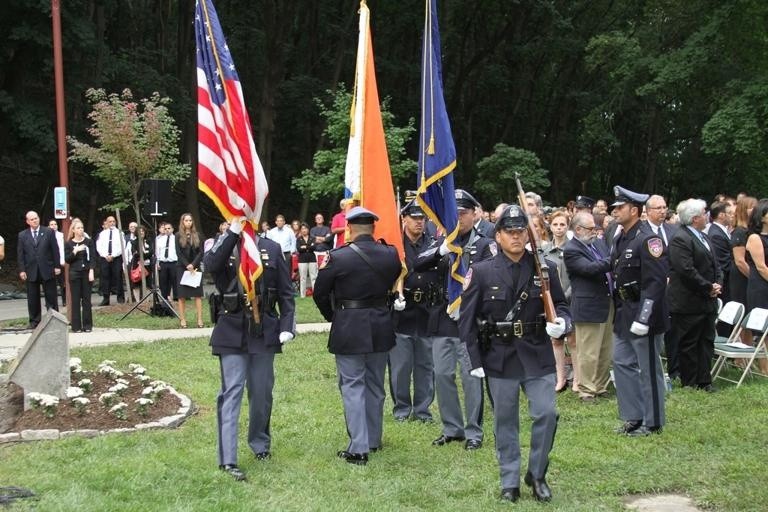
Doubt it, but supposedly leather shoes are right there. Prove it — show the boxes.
[99,300,109,306]
[626,425,663,437]
[613,419,642,434]
[27,323,37,329]
[337,450,368,466]
[524,470,552,502]
[257,452,271,462]
[465,439,482,451]
[496,486,520,504]
[432,434,465,446]
[220,465,244,480]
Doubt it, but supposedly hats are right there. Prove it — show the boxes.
[454,189,479,210]
[404,190,417,204]
[494,205,528,232]
[345,206,379,224]
[609,185,649,206]
[403,200,426,217]
[573,196,595,209]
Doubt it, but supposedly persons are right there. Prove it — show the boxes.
[730,197,758,369]
[413,189,497,450]
[157,224,179,302]
[125,222,139,244]
[473,204,496,240]
[460,205,575,504]
[47,220,66,306]
[267,214,296,273]
[665,210,677,224]
[64,221,96,333]
[744,198,767,375]
[543,210,579,392]
[204,220,296,480]
[297,223,318,298]
[17,211,61,330]
[525,190,541,216]
[596,200,613,222]
[727,205,737,237]
[388,199,436,427]
[527,216,548,251]
[96,216,126,306]
[175,213,204,328]
[0,234,6,258]
[313,207,402,466]
[260,222,271,239]
[610,185,667,438]
[737,191,748,204]
[292,220,300,235]
[332,199,346,249]
[310,213,331,255]
[668,198,724,395]
[155,222,165,238]
[705,194,725,223]
[642,195,678,246]
[708,202,735,338]
[130,226,152,302]
[576,196,595,215]
[563,212,614,405]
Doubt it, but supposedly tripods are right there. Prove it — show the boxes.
[120,217,181,323]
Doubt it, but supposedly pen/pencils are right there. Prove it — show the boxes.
[193,268,198,275]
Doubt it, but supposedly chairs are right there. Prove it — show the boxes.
[712,308,768,389]
[708,301,746,375]
[714,298,722,325]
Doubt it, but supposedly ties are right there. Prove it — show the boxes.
[165,236,169,258]
[108,230,112,256]
[33,231,38,245]
[588,243,614,297]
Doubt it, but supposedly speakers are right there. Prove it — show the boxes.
[142,179,172,219]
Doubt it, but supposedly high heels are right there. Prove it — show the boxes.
[554,379,568,393]
[180,318,187,328]
[197,319,204,328]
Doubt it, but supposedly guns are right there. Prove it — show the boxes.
[514,172,559,324]
[394,185,404,301]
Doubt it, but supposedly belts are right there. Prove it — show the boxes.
[614,288,629,302]
[488,321,536,336]
[333,295,387,309]
[403,288,426,303]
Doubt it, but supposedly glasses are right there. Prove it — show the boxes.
[579,225,593,230]
[650,207,668,211]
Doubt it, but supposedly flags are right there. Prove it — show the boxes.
[195,0,269,324]
[344,0,408,295]
[417,1,466,314]
